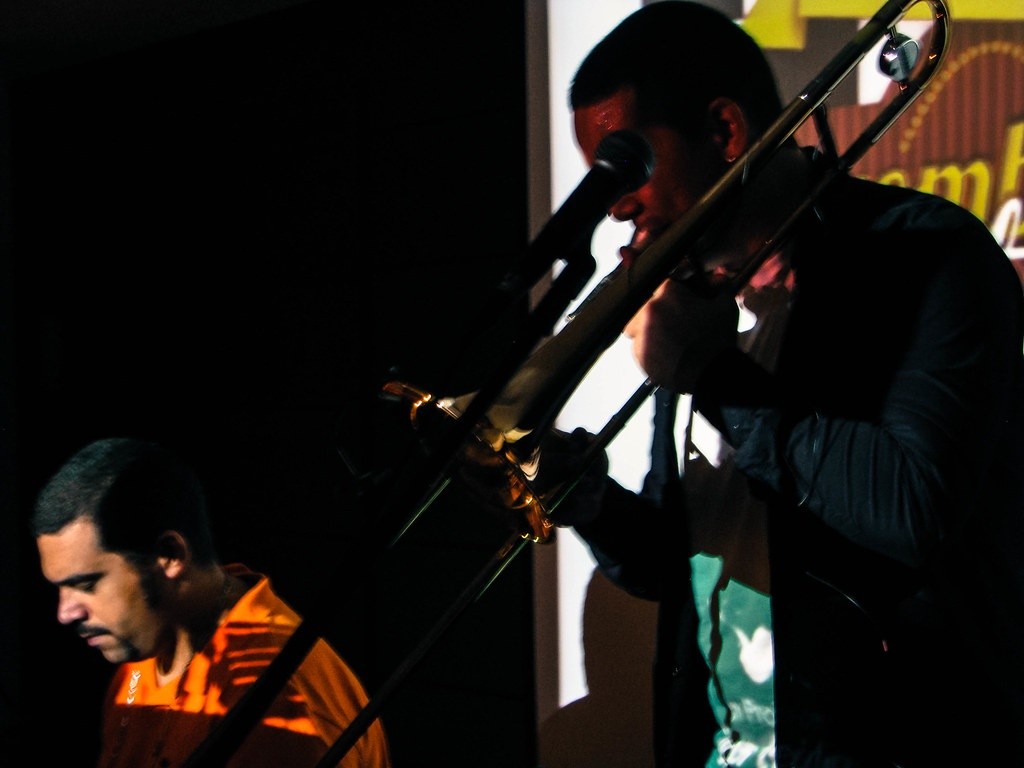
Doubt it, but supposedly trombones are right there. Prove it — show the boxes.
[183,0,955,768]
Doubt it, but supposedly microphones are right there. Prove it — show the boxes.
[500,129,657,304]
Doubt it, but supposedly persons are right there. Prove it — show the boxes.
[35,439,389,767]
[536,0,1024,768]
